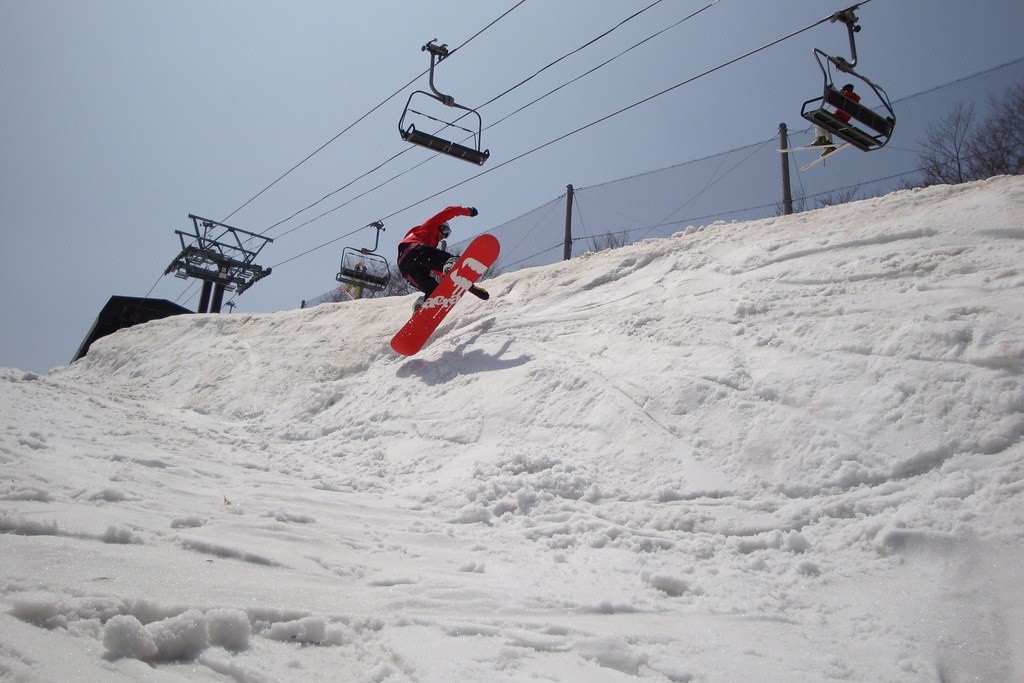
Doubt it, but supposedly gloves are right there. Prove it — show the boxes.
[468,207,478,217]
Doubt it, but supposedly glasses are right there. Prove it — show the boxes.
[440,224,452,238]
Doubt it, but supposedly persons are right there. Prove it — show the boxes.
[396,207,489,311]
[345,261,363,291]
[807,84,861,157]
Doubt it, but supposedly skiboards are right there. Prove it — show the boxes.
[775,142,851,171]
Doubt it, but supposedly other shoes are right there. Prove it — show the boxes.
[443,257,459,276]
[412,296,425,312]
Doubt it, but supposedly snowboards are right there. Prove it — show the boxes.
[391,233,502,357]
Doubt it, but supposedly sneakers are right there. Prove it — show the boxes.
[820,147,836,157]
[810,136,826,146]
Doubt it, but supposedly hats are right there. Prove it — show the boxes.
[442,221,450,229]
[842,84,854,93]
